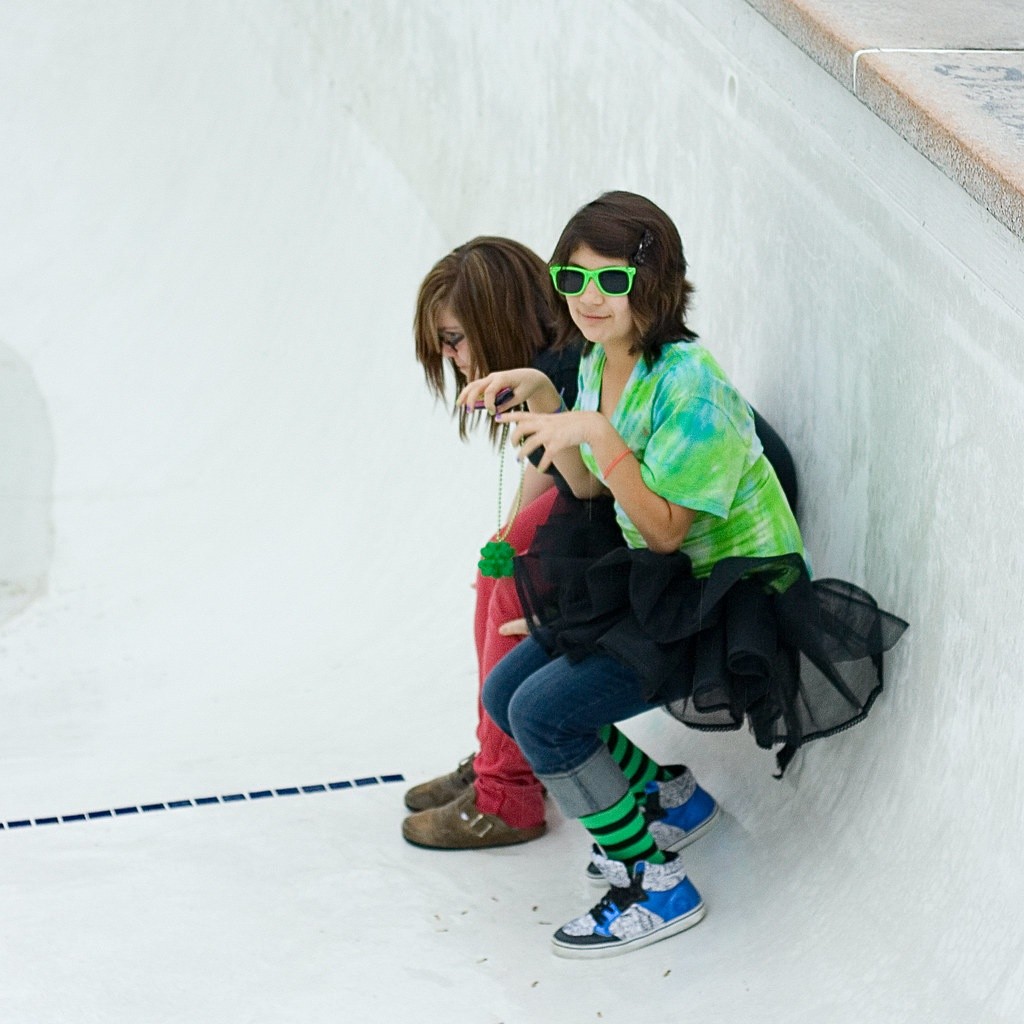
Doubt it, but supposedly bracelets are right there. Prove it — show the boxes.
[597,445,638,484]
[548,391,567,427]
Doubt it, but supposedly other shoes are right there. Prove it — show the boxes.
[401,753,549,852]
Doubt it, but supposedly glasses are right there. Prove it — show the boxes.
[440,335,465,353]
[550,264,636,296]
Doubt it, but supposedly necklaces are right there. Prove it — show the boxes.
[476,387,533,582]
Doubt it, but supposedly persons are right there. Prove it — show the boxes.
[453,186,815,962]
[396,231,803,856]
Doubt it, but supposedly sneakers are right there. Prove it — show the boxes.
[551,849,705,957]
[583,764,721,888]
